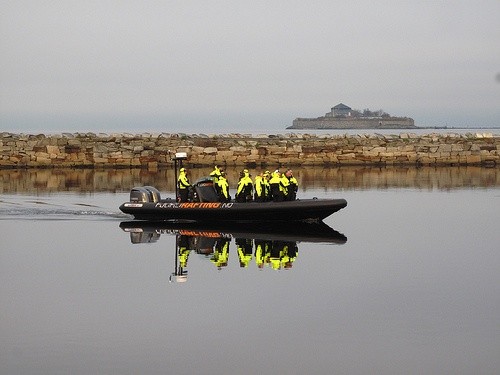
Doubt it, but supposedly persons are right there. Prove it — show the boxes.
[177,168,189,202]
[208,164,299,201]
[178,235,191,268]
[209,237,299,270]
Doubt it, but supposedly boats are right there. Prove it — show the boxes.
[119,152,348,221]
